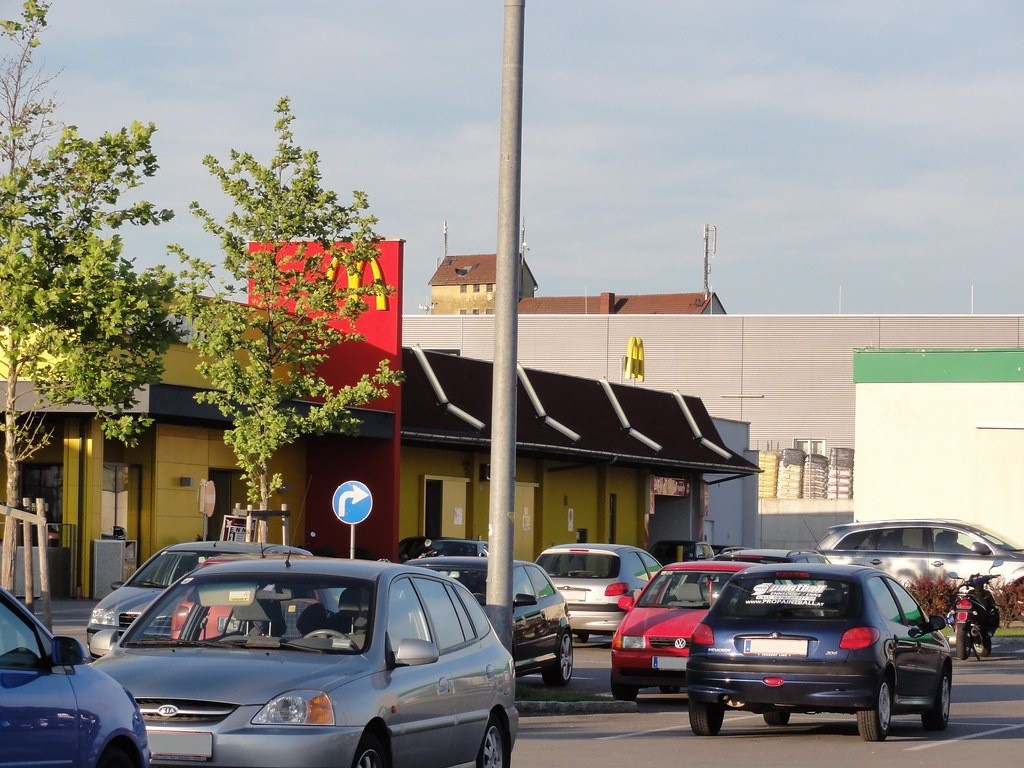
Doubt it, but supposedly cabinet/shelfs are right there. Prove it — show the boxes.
[93,539,137,600]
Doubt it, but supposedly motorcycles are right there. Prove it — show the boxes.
[948,558,1004,661]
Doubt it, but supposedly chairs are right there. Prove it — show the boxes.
[674,583,703,602]
[339,587,372,635]
[822,590,840,604]
[219,590,281,639]
[570,559,586,571]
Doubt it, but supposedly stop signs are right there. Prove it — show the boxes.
[204,481,215,518]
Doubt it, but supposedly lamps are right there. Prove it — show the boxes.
[180,477,194,486]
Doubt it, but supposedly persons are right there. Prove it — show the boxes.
[938,528,971,552]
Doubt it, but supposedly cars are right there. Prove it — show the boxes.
[171,551,326,641]
[685,563,955,742]
[604,561,766,701]
[814,518,1024,622]
[398,536,488,559]
[646,539,713,561]
[88,556,518,768]
[86,541,338,648]
[402,557,573,686]
[0,588,154,768]
[709,544,751,554]
[522,543,663,641]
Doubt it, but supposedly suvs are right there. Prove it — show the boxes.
[697,547,832,603]
